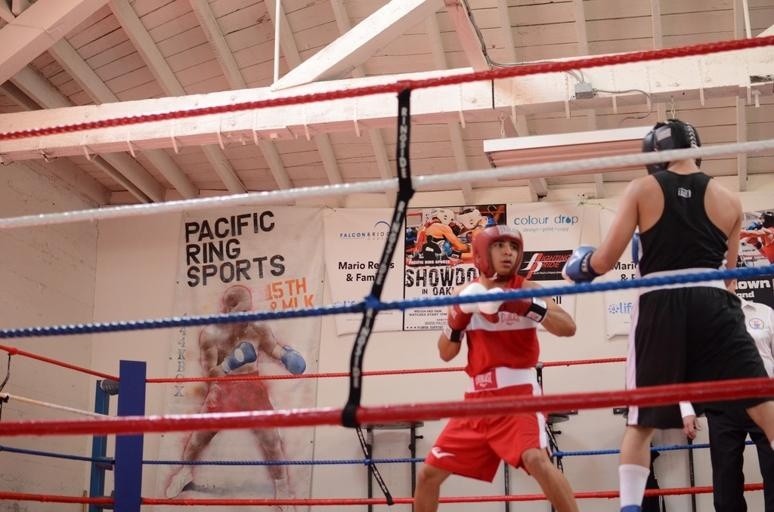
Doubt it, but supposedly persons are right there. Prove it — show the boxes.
[738,210,774,268]
[406,205,505,264]
[677,264,773,512]
[564,118,774,512]
[406,224,580,511]
[165,286,305,512]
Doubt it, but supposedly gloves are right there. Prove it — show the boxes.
[746,236,758,244]
[279,345,307,375]
[561,246,601,284]
[224,341,258,370]
[746,221,758,230]
[443,282,488,343]
[478,286,549,324]
[740,230,747,240]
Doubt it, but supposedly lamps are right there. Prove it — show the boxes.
[482,95,676,183]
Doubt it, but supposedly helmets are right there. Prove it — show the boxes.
[457,207,482,230]
[642,118,702,176]
[472,224,524,282]
[758,211,774,227]
[433,209,455,226]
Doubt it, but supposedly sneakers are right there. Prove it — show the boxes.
[275,494,296,512]
[165,471,194,499]
